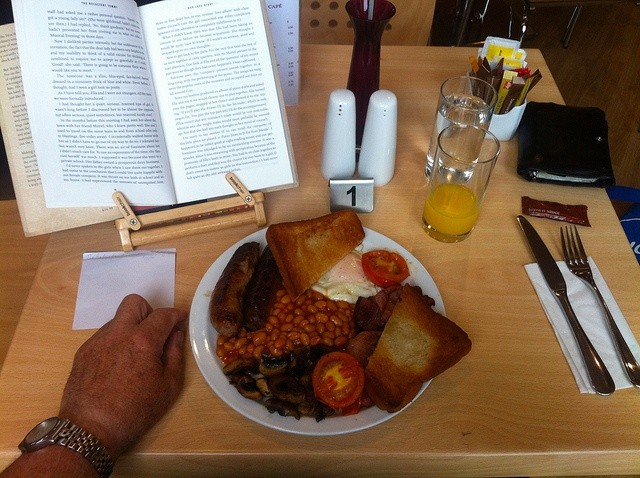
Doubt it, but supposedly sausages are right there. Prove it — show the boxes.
[239,242,283,333]
[208,243,261,339]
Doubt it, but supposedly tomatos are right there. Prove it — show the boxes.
[358,247,411,288]
[312,351,364,409]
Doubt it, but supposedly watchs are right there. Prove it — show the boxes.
[18,417,114,478]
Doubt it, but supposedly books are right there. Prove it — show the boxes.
[517,102,616,188]
[12,0,299,210]
[0,22,164,237]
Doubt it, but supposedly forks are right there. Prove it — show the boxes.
[560,225,640,389]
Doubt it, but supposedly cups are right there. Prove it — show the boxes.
[420,122,500,244]
[484,98,530,141]
[423,75,498,186]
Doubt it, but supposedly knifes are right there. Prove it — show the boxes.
[516,214,615,396]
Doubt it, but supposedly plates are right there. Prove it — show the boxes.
[188,225,446,438]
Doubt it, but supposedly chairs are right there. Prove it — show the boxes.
[431,0,492,48]
[502,0,587,49]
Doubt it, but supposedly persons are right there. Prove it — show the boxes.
[0,294,187,478]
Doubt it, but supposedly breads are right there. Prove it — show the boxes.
[265,209,366,303]
[362,283,474,410]
